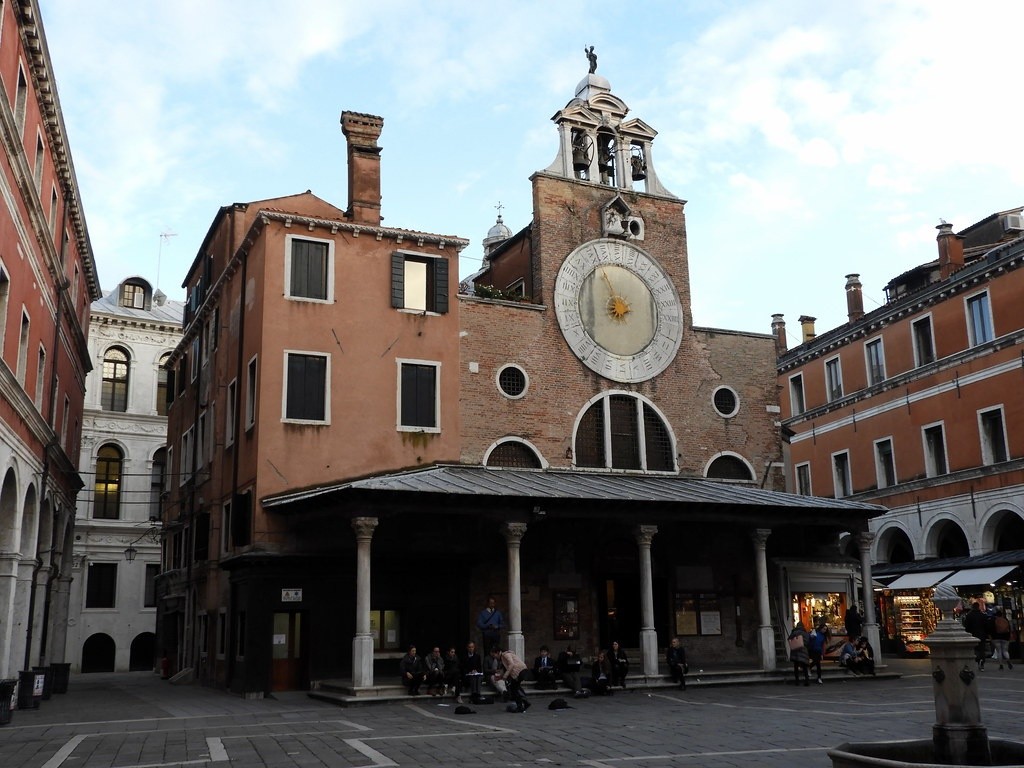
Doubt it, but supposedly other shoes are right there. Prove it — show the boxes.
[818,678,823,684]
[999,665,1003,669]
[408,691,415,695]
[807,666,812,677]
[456,696,464,704]
[1009,664,1013,670]
[981,668,985,672]
[415,691,420,694]
[524,702,530,710]
[452,686,456,695]
[428,689,436,695]
[439,690,443,695]
[513,706,524,713]
[979,660,983,669]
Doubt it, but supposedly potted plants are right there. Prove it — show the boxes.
[518,296,532,304]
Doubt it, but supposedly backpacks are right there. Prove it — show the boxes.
[995,615,1010,634]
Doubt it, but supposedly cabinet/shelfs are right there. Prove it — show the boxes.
[896,598,923,631]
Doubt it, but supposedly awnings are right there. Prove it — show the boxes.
[883,566,1017,589]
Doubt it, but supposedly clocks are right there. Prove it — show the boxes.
[553,237,683,384]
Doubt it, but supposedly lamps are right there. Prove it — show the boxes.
[125,527,158,565]
[566,447,573,461]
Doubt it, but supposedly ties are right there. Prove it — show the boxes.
[543,659,545,667]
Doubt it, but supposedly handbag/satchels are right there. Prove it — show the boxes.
[788,630,804,651]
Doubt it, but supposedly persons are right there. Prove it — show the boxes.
[789,621,830,685]
[965,603,1013,672]
[534,645,589,698]
[586,46,597,73]
[841,604,875,675]
[400,598,531,713]
[592,641,629,695]
[666,638,688,691]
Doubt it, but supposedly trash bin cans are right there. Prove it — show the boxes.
[18,670,46,709]
[0,679,19,724]
[32,667,56,700]
[50,662,71,694]
